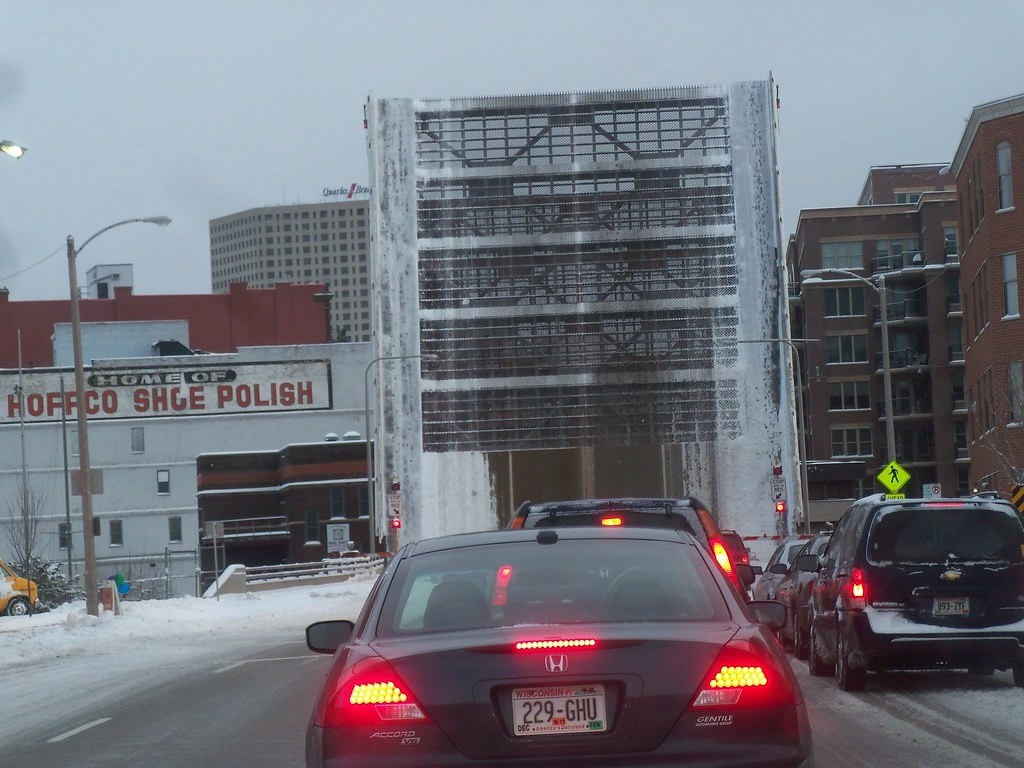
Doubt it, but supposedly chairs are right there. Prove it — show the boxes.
[422,564,697,624]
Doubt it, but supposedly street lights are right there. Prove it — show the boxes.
[364,354,441,552]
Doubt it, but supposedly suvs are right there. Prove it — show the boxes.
[809,492,1024,692]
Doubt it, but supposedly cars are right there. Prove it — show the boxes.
[0,559,38,617]
[509,496,765,625]
[752,531,836,660]
[68,215,171,618]
[305,528,814,768]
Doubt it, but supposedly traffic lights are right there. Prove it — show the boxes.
[390,481,400,491]
[391,519,399,527]
